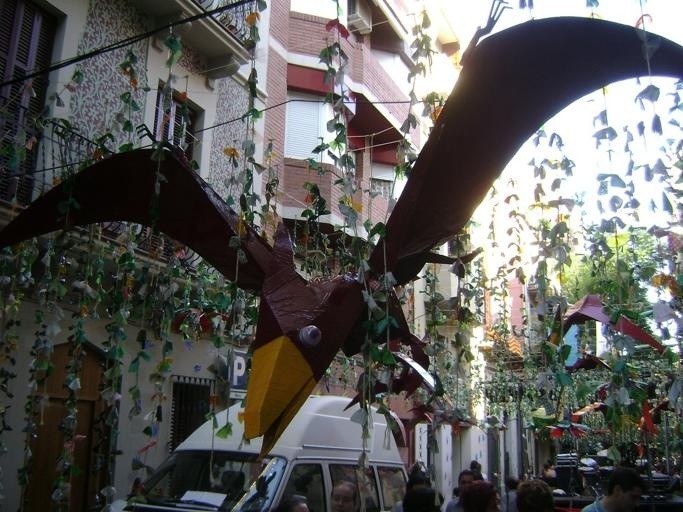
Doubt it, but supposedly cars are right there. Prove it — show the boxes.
[555,452,681,488]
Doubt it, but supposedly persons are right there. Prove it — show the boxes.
[581,467,648,512]
[330,481,361,512]
[270,495,311,512]
[391,460,557,512]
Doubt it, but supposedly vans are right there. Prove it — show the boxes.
[123,395,409,512]
